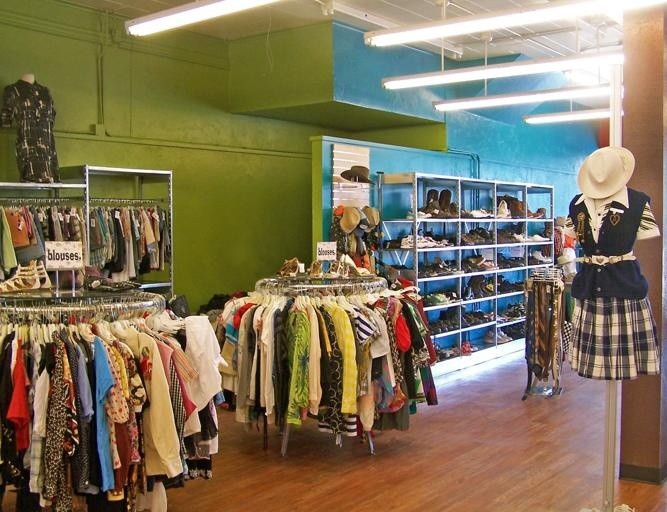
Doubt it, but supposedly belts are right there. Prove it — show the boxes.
[521,281,560,401]
[584,249,637,266]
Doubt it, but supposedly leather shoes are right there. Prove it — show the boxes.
[83,274,142,292]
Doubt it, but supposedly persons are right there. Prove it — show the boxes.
[1,72,61,183]
[567,145,660,381]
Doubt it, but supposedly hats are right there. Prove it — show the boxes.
[577,145,636,200]
[341,165,376,184]
[554,216,567,231]
[339,206,380,234]
[556,255,573,265]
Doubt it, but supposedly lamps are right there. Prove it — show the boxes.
[361,0,624,125]
[124,0,277,38]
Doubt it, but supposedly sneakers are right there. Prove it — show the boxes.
[383,227,551,249]
[400,251,553,359]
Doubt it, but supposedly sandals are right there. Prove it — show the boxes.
[0,260,53,294]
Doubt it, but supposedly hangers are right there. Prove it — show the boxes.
[0,197,69,211]
[0,298,185,346]
[254,279,366,310]
[90,197,158,211]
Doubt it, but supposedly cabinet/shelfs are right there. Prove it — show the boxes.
[376,171,556,381]
[0,163,175,303]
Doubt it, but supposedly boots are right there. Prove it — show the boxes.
[406,190,546,219]
